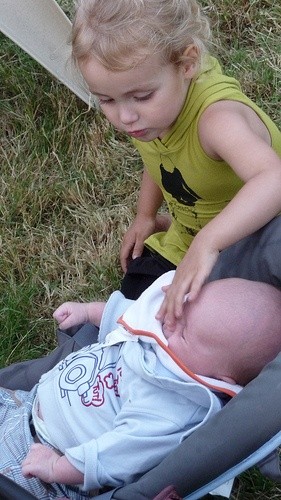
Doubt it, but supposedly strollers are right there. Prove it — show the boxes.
[0,211,281,500]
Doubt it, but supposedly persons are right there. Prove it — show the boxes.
[66,0,281,328]
[1,276,281,499]
